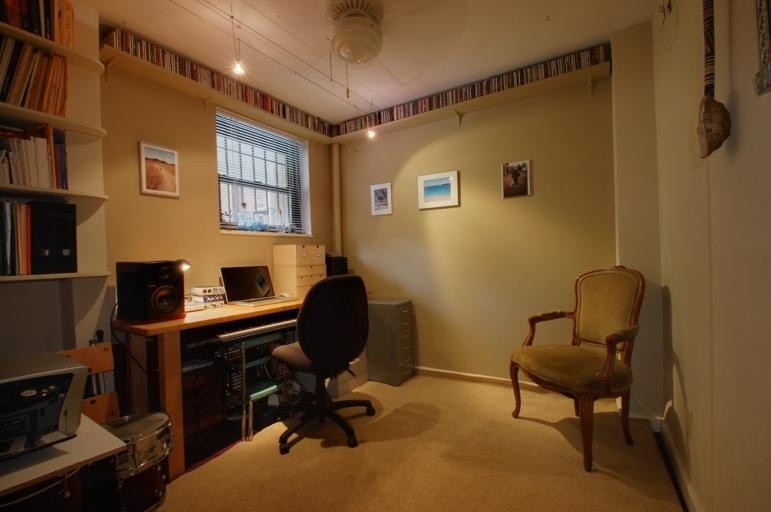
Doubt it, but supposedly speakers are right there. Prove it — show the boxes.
[116,260,185,325]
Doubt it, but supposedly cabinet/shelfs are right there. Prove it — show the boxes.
[367,297,414,387]
[274,243,325,296]
[1,0,113,403]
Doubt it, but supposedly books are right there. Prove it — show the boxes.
[105,26,332,137]
[338,44,606,136]
[2,1,78,275]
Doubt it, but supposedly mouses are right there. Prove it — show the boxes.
[280,293,290,298]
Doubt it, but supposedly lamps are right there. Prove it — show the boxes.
[176,257,192,272]
[325,1,386,66]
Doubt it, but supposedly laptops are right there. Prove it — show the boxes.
[220,265,291,307]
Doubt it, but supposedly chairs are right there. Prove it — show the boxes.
[270,272,378,451]
[506,263,645,472]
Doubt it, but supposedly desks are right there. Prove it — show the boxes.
[0,412,129,512]
[111,294,304,480]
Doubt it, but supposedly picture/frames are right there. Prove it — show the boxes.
[371,183,394,216]
[417,170,459,211]
[139,141,180,198]
